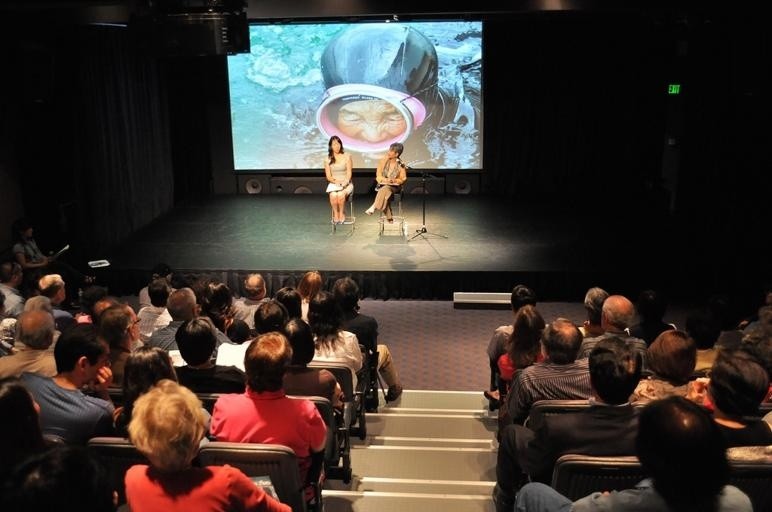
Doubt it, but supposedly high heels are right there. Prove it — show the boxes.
[339,214,346,224]
[364,206,375,216]
[387,210,393,224]
[332,215,339,225]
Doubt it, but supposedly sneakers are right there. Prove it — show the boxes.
[484,391,500,403]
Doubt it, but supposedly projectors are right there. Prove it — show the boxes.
[159,0,251,59]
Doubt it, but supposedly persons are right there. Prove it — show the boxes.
[2,262,25,317]
[512,394,751,511]
[36,275,73,330]
[324,137,354,225]
[274,287,303,319]
[13,324,118,484]
[74,286,107,322]
[714,289,772,359]
[298,272,322,326]
[1,384,71,511]
[308,291,363,400]
[13,221,81,308]
[167,270,188,289]
[631,329,709,407]
[487,285,550,367]
[89,299,145,351]
[199,282,251,344]
[702,352,772,447]
[232,274,272,334]
[138,264,176,309]
[210,331,328,495]
[5,443,122,511]
[496,319,595,422]
[492,336,648,511]
[332,277,403,413]
[278,317,346,428]
[366,141,407,224]
[123,379,291,511]
[241,301,291,371]
[135,279,172,344]
[22,296,62,348]
[0,309,58,378]
[146,288,234,357]
[484,305,546,402]
[579,288,629,339]
[630,290,677,346]
[193,277,207,305]
[111,345,208,439]
[90,304,142,387]
[174,317,247,395]
[1,293,20,355]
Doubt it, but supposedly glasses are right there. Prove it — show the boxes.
[132,318,141,326]
[10,261,17,276]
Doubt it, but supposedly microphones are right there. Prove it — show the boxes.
[396,159,404,168]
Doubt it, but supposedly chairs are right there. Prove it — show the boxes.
[196,442,325,511]
[85,387,127,407]
[727,445,772,512]
[284,393,353,484]
[550,452,644,502]
[85,435,146,506]
[359,344,378,414]
[41,433,67,449]
[306,359,371,442]
[193,391,224,417]
[522,398,591,440]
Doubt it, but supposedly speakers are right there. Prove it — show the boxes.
[446,169,489,195]
[272,172,446,194]
[236,171,270,195]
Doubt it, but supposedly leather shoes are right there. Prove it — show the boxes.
[387,385,403,401]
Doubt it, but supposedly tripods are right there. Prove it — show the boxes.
[407,168,448,242]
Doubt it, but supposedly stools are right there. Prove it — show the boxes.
[376,182,406,241]
[330,181,356,235]
